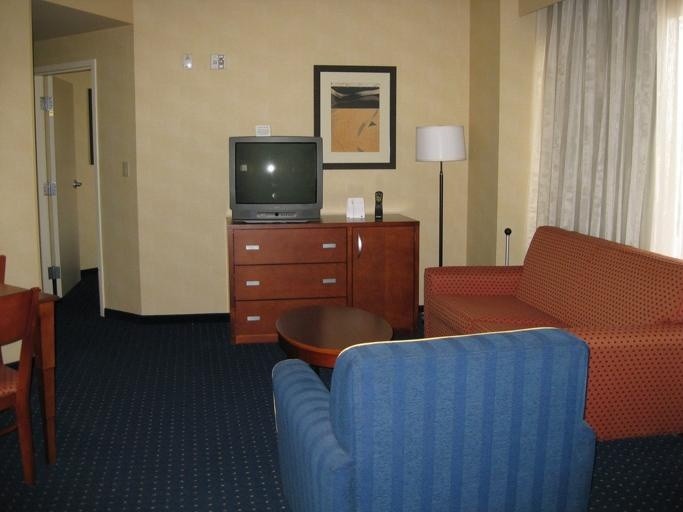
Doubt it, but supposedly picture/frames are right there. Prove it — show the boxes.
[313,64,396,170]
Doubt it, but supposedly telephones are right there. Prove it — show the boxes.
[375,191,383,222]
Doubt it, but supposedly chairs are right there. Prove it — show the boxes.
[0,286,41,484]
[0,255,7,284]
[271,326,598,511]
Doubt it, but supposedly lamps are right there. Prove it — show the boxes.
[415,126,466,267]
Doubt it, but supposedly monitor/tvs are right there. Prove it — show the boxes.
[228,136,324,224]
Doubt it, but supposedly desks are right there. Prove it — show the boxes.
[0,283,61,465]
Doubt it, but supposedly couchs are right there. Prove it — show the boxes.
[425,224,683,441]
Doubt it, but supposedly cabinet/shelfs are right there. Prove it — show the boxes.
[225,212,420,344]
